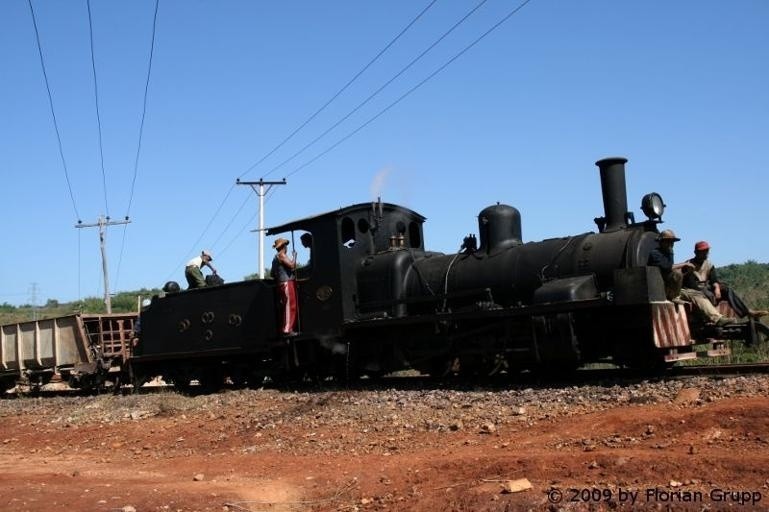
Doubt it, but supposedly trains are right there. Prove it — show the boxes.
[1,156,738,397]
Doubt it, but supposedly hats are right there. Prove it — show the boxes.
[201,250,215,261]
[272,238,290,249]
[694,241,712,253]
[654,229,680,242]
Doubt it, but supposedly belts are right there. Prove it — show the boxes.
[186,265,197,269]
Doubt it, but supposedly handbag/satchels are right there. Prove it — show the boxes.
[204,274,224,287]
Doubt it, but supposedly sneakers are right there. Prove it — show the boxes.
[281,329,297,336]
[716,316,736,326]
[747,308,769,319]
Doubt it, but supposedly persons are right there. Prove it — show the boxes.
[647,229,738,328]
[184,249,216,290]
[272,237,303,337]
[681,241,768,320]
